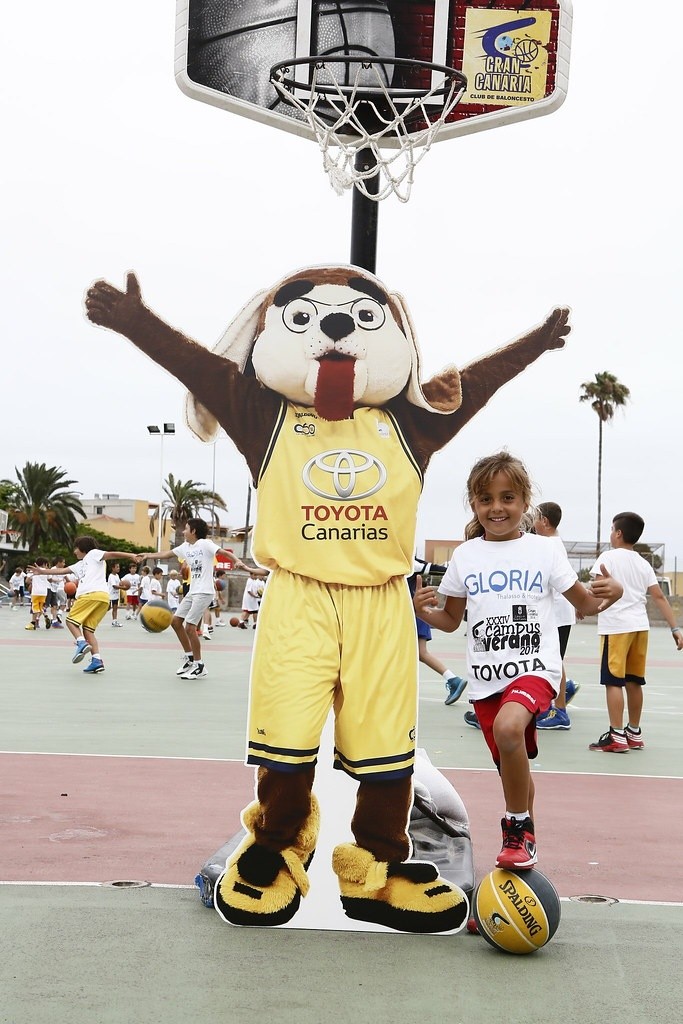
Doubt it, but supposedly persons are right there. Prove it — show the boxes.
[0,554,265,640]
[130,518,270,680]
[412,452,624,936]
[575,511,683,753]
[25,536,144,673]
[405,502,582,731]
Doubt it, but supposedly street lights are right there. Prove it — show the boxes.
[147,423,175,568]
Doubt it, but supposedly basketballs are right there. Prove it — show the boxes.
[471,865,562,954]
[229,617,240,626]
[139,599,174,634]
[176,585,184,594]
[214,579,225,591]
[121,580,131,590]
[64,582,78,594]
[258,588,264,596]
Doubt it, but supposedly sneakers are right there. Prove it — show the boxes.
[467,919,480,934]
[464,711,481,729]
[536,706,571,729]
[494,816,537,869]
[72,641,92,663]
[589,726,629,752]
[83,657,105,672]
[565,680,580,705]
[176,655,208,680]
[624,723,644,748]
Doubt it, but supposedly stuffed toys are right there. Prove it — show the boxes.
[82,259,571,933]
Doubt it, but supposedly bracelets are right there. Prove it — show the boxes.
[671,626,680,631]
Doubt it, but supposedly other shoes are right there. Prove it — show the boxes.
[45,618,51,628]
[215,621,226,627]
[52,622,64,628]
[445,677,467,705]
[238,622,248,629]
[10,605,17,610]
[112,621,123,627]
[203,631,211,640]
